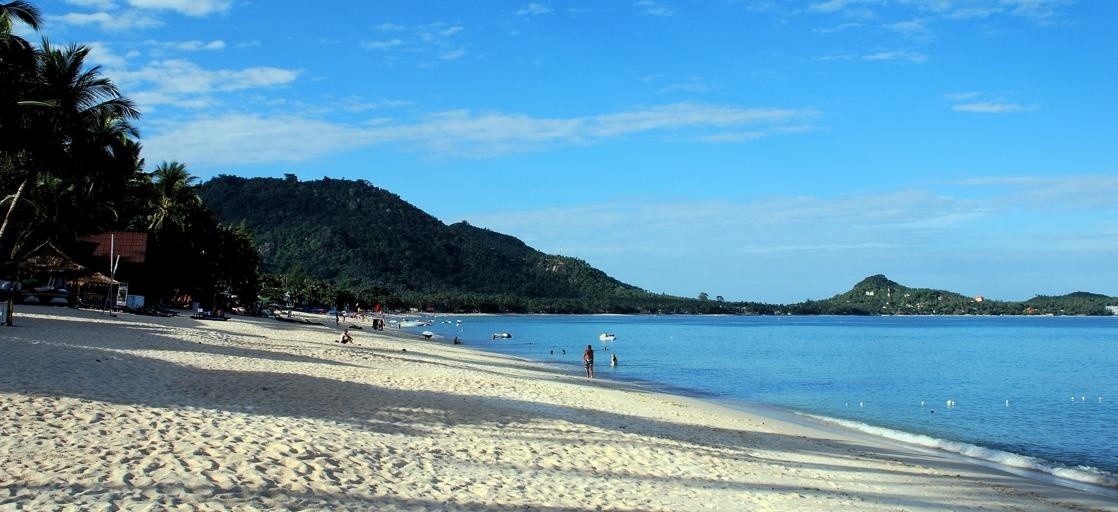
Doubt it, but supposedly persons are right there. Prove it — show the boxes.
[610,354,617,366]
[583,344,593,379]
[454,337,461,344]
[340,329,353,344]
[335,311,341,327]
